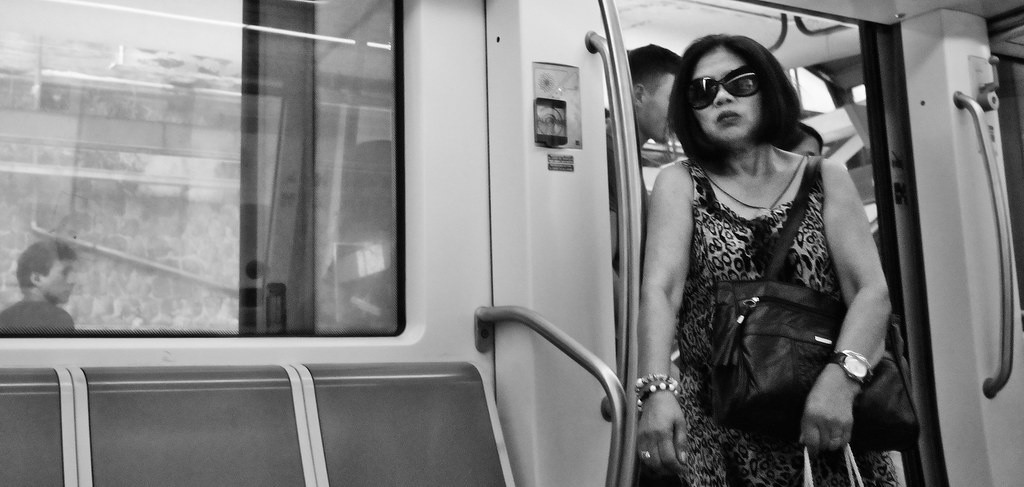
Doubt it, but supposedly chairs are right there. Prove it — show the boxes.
[0,367,77,487]
[293,359,518,487]
[67,365,317,487]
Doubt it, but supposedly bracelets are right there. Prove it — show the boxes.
[842,349,872,368]
[634,373,684,418]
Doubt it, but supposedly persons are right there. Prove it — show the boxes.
[637,34,901,487]
[0,239,78,329]
[776,121,825,159]
[605,43,681,348]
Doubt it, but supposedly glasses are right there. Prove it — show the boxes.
[687,72,760,109]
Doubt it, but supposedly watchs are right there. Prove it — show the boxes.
[818,352,875,385]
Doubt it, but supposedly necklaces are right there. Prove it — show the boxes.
[697,154,806,210]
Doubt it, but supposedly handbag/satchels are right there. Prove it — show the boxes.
[710,156,922,449]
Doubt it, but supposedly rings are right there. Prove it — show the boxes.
[640,449,650,461]
